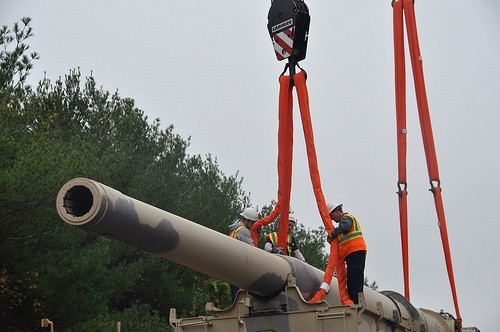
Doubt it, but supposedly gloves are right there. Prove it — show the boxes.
[271,247,286,255]
[327,234,334,244]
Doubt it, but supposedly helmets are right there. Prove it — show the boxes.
[288,213,297,223]
[326,202,343,215]
[240,208,258,221]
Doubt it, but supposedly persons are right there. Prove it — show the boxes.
[325,201,368,303]
[228,207,259,305]
[264,213,309,264]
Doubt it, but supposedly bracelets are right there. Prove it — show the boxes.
[327,234,332,239]
